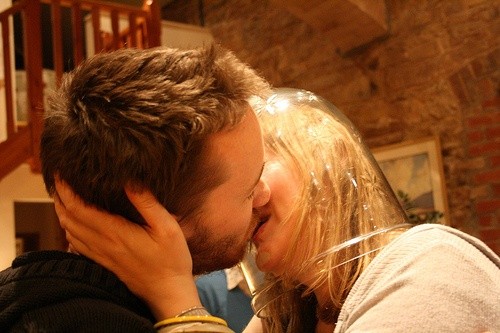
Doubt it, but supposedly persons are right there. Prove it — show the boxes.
[1,43,270,332]
[55,87,500,333]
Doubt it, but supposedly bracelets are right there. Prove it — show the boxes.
[152,306,227,329]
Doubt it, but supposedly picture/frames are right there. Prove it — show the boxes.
[370,135,451,228]
